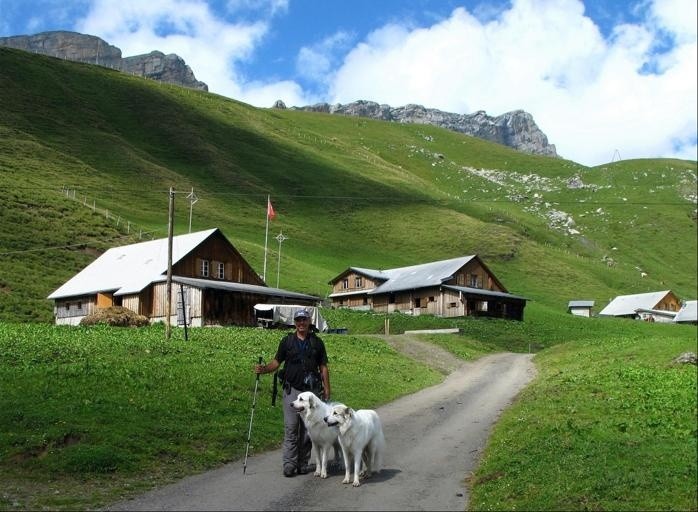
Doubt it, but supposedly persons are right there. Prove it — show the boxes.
[254,311,330,477]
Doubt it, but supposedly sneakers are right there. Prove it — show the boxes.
[284,467,295,477]
[298,465,308,474]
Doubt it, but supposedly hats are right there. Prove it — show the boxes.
[294,310,310,319]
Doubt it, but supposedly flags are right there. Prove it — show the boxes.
[267,201,275,221]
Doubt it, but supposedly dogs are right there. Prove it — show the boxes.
[290,391,357,479]
[323,403,384,486]
